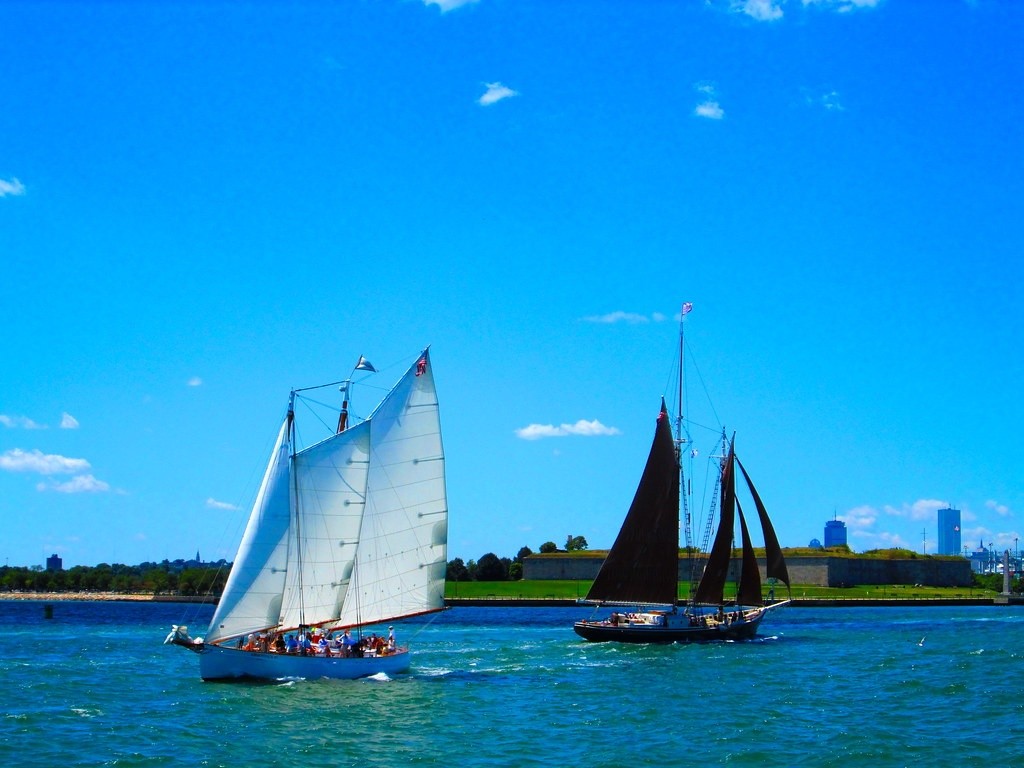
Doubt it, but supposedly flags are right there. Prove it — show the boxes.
[682,302,693,315]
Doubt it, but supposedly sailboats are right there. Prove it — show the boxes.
[164,343,453,681]
[574,302,792,645]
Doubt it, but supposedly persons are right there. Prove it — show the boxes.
[241,625,397,658]
[683,607,689,616]
[730,611,744,624]
[581,611,639,626]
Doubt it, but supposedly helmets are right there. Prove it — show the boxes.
[389,626,394,628]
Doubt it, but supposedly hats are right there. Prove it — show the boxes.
[321,635,325,638]
